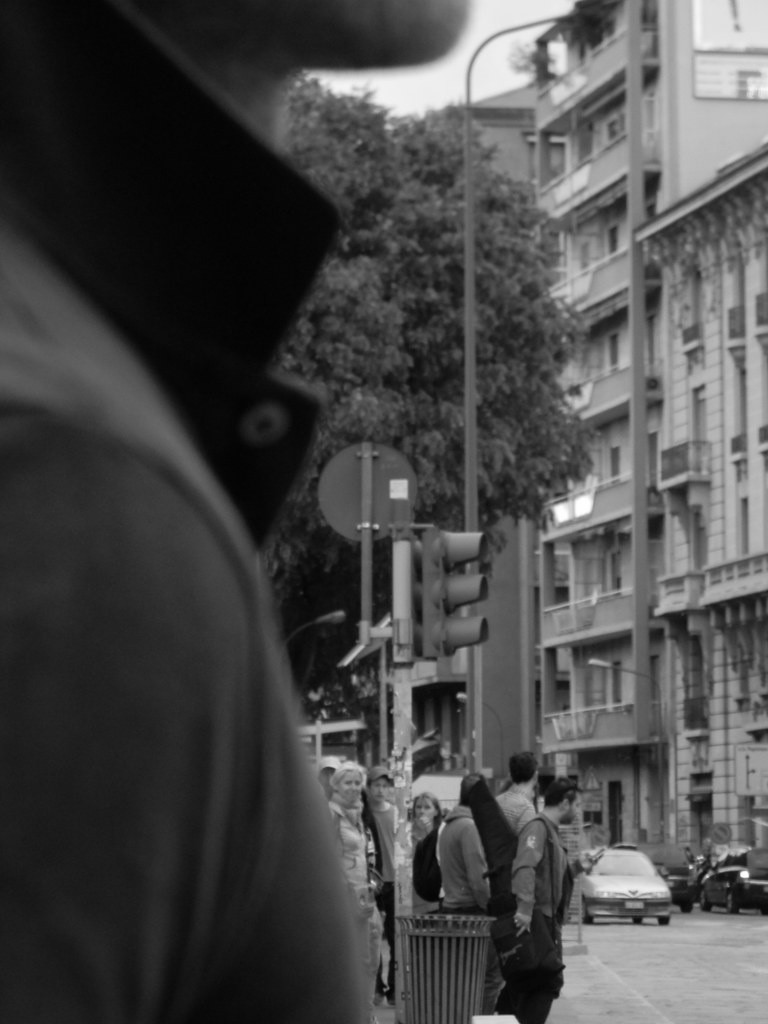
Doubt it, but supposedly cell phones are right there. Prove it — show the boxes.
[590,848,606,862]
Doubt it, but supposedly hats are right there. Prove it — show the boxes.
[366,766,389,781]
[318,756,340,772]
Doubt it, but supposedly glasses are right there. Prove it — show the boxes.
[570,778,578,803]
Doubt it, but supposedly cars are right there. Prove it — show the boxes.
[581,849,673,924]
[699,844,768,917]
[654,862,695,913]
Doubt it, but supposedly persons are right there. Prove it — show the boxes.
[0,0,474,1024]
[328,760,388,1024]
[506,778,604,1023]
[410,793,442,916]
[359,763,401,1007]
[315,765,337,801]
[494,752,539,836]
[438,772,508,1014]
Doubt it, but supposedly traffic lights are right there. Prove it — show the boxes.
[423,525,490,657]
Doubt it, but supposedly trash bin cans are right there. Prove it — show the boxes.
[394,914,498,1024]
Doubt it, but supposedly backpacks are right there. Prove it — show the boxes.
[413,821,444,902]
[460,776,565,1005]
